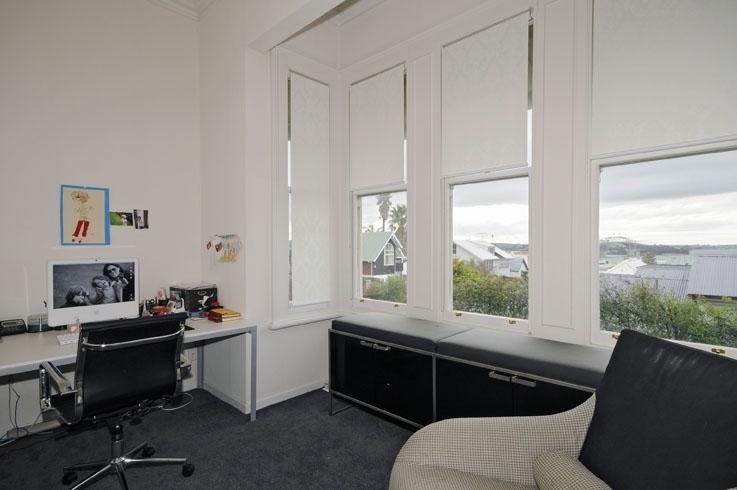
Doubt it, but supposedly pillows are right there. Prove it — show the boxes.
[580,328,736,490]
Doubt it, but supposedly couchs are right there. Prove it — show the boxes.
[388,329,736,490]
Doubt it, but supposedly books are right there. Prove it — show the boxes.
[207,305,240,324]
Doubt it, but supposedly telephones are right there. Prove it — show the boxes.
[0,319,26,337]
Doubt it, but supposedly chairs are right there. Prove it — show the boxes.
[39,312,195,489]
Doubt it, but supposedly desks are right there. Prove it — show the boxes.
[0,317,257,421]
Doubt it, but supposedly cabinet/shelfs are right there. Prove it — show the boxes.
[328,314,470,432]
[434,329,614,421]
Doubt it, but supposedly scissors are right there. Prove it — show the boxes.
[145,299,155,305]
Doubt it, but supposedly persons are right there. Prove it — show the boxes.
[59,286,87,307]
[70,191,96,243]
[103,264,134,302]
[86,272,128,306]
